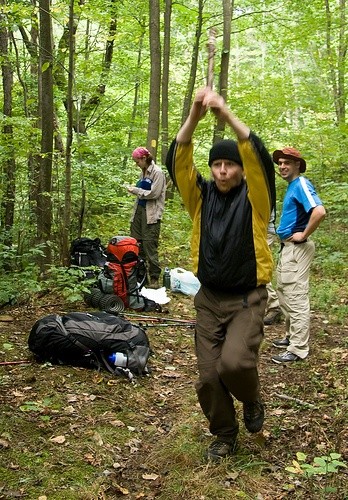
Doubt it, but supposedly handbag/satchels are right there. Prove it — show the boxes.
[68,236,108,267]
[170,267,202,297]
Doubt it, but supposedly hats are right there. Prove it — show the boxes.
[273,147,306,173]
[208,139,243,168]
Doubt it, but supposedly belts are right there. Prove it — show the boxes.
[279,238,308,253]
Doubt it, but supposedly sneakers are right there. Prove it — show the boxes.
[208,440,239,459]
[272,336,290,348]
[243,399,264,433]
[263,307,284,325]
[271,351,301,364]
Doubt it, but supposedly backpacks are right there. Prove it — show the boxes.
[28,312,150,374]
[98,236,146,310]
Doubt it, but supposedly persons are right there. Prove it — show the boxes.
[165,86,275,460]
[271,148,326,363]
[126,147,167,288]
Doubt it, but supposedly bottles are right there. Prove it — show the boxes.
[163,267,171,288]
[109,352,145,369]
